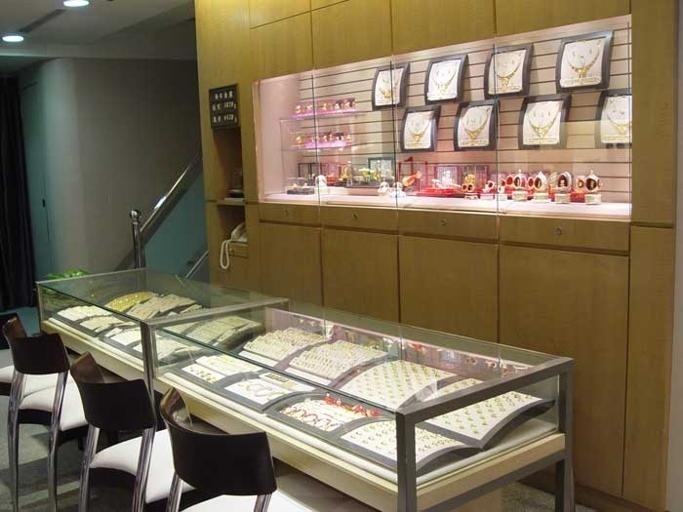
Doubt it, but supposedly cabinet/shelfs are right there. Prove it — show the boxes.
[259,202,399,323]
[142,299,577,512]
[399,209,631,498]
[33,265,289,406]
[246,0,631,80]
[200,124,255,295]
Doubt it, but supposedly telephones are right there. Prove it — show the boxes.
[231,222,248,243]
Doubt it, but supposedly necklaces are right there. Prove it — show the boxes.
[104,290,382,433]
[378,71,401,98]
[566,45,600,78]
[606,96,632,136]
[431,65,458,91]
[495,56,521,87]
[407,114,431,142]
[462,107,489,139]
[527,103,562,138]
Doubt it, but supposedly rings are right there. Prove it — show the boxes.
[347,360,532,457]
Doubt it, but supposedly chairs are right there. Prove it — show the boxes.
[1,312,19,401]
[58,351,202,512]
[158,383,355,511]
[0,317,91,512]
